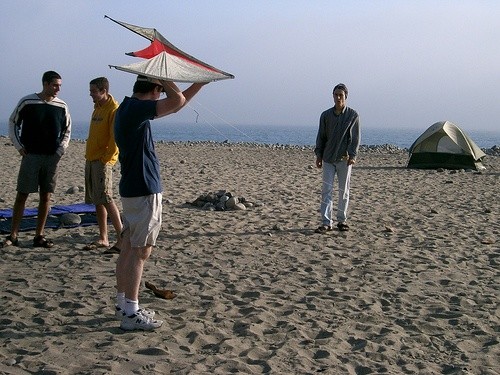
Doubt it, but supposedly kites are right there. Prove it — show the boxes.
[104,14,235,83]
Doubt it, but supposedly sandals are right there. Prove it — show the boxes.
[33,235,54,248]
[315,225,332,233]
[337,222,349,231]
[0,236,18,248]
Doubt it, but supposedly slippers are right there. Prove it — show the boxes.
[103,246,121,254]
[85,241,111,250]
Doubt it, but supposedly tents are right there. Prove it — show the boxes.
[406,121,486,170]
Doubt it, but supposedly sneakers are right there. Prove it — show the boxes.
[114,303,156,321]
[120,309,163,330]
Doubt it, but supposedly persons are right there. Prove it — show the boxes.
[0,71,72,249]
[314,84,360,234]
[114,74,210,331]
[83,77,122,255]
